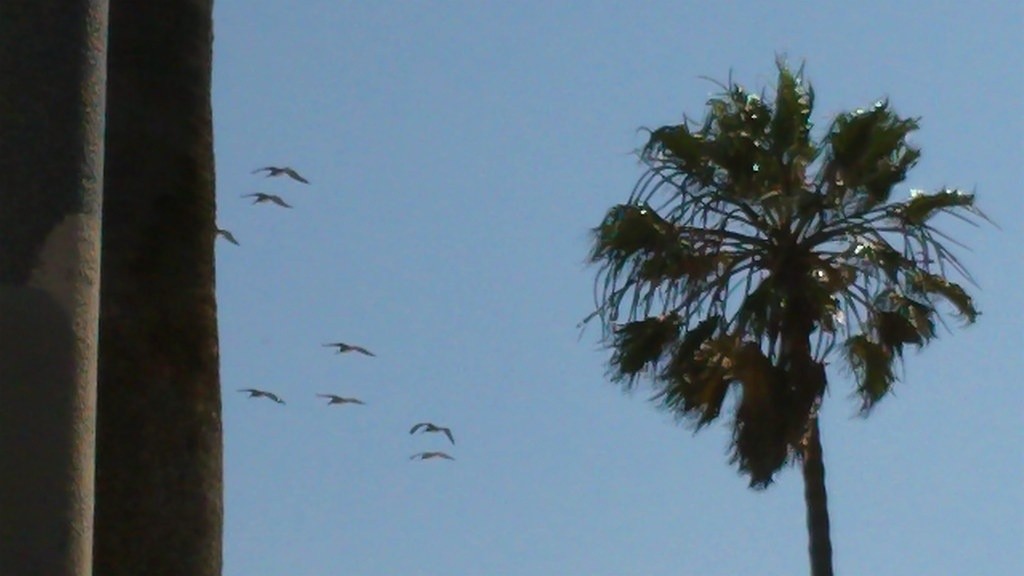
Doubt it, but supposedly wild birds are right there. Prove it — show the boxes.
[238,341,455,462]
[216,165,310,246]
